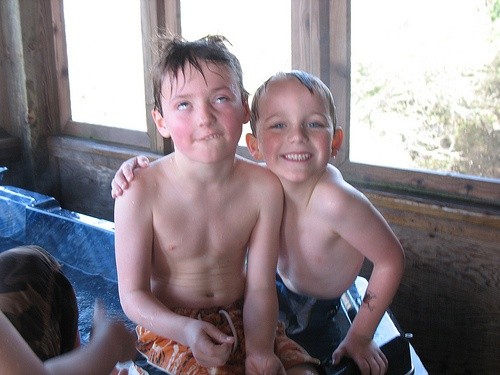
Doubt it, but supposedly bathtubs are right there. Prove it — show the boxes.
[0,165,431,375]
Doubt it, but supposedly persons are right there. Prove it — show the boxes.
[0,244,139,375]
[110,69,406,375]
[113,27,321,375]
